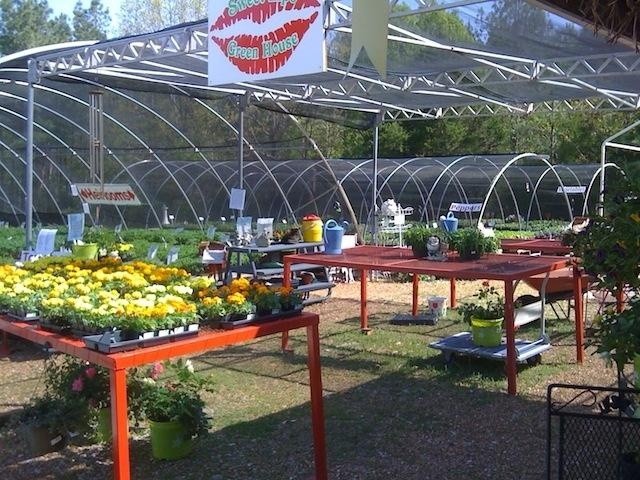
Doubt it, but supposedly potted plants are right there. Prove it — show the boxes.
[402,223,447,257]
[449,228,498,260]
[453,281,522,347]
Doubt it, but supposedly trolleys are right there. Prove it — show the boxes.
[427,271,552,377]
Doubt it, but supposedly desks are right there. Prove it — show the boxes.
[500,238,584,256]
[0,309,329,480]
[282,245,584,397]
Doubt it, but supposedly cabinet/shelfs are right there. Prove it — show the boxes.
[225,241,334,301]
[545,354,640,480]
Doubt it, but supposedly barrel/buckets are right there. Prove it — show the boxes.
[301,213,323,242]
[147,419,193,461]
[71,242,100,263]
[94,406,113,444]
[470,315,505,349]
[429,296,448,318]
